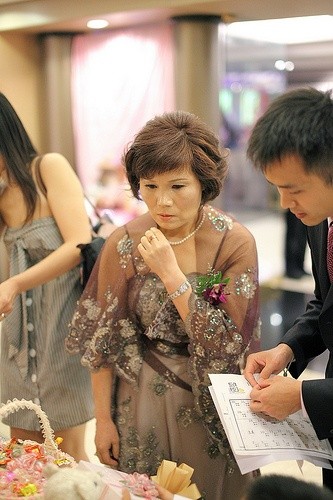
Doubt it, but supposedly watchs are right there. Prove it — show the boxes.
[167,280,189,303]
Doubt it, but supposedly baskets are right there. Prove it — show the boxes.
[0,397,77,500]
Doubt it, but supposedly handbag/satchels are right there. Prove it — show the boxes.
[76,213,117,271]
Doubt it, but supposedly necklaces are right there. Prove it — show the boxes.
[155,208,205,247]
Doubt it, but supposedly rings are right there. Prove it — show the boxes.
[147,235,155,242]
[1,313,5,319]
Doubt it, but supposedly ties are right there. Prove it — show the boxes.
[326,225,333,286]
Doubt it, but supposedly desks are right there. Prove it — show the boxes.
[0,439,194,499]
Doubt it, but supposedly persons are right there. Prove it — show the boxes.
[0,94,98,467]
[241,87,333,495]
[233,474,332,500]
[64,112,261,500]
